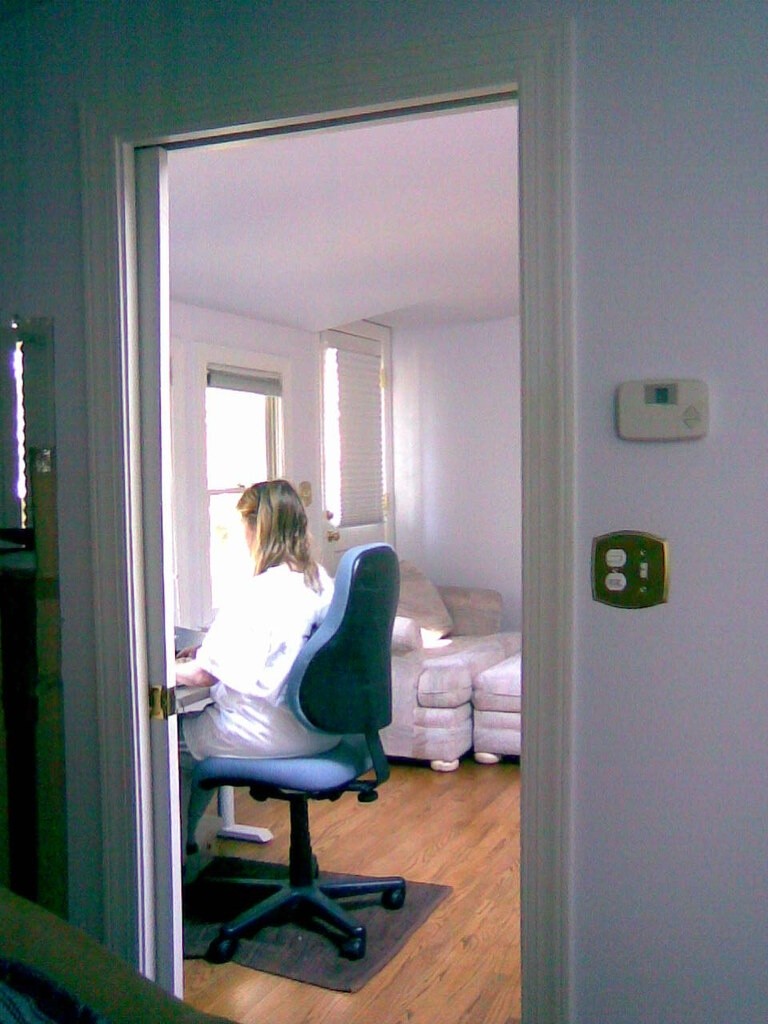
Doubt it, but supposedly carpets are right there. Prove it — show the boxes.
[182,856,454,995]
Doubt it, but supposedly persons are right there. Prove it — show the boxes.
[174,479,336,853]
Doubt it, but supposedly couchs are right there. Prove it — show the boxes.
[379,584,521,772]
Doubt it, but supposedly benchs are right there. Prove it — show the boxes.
[471,650,521,764]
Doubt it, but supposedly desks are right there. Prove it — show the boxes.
[174,685,275,843]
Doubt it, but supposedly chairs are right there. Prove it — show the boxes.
[191,541,407,965]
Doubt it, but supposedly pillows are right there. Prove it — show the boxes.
[395,560,454,643]
